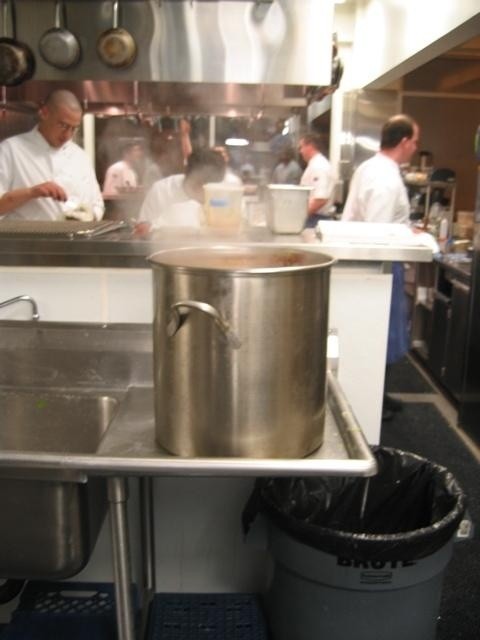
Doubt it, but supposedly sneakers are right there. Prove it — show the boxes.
[382,395,403,422]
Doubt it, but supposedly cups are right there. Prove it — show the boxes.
[271,182,311,234]
[203,179,244,237]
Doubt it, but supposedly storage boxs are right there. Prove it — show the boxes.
[8,580,138,640]
[142,592,268,640]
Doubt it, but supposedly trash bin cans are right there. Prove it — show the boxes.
[253,446,469,640]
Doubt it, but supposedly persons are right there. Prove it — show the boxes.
[0,87,106,223]
[137,146,226,231]
[298,131,339,230]
[340,113,423,423]
[103,141,141,197]
[270,150,304,186]
[213,144,241,184]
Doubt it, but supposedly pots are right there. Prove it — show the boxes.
[145,242,339,457]
[39,1,81,68]
[0,2,36,87]
[97,1,137,71]
